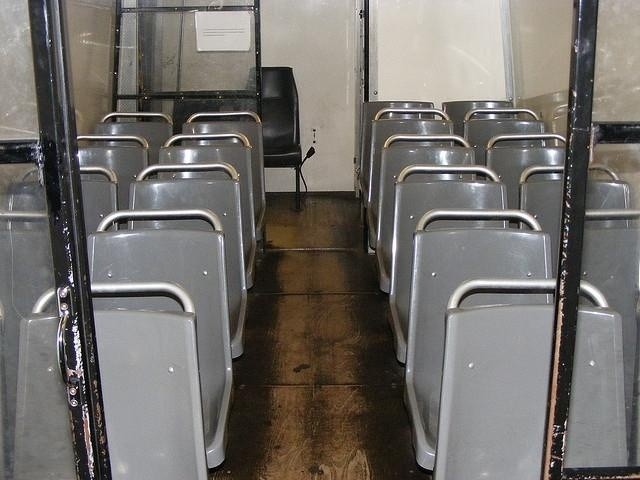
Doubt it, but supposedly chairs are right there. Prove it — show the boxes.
[248,66,303,212]
